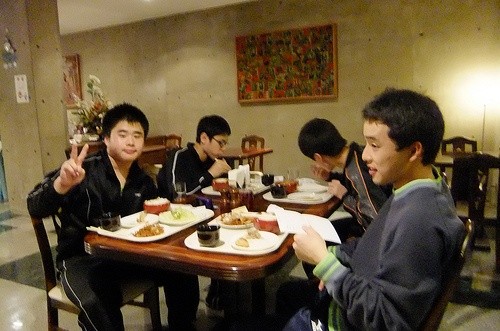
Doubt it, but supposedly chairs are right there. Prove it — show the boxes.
[30,205,162,331]
[441,136,477,172]
[150,135,181,148]
[142,145,166,164]
[423,217,475,331]
[241,134,264,173]
[164,148,182,202]
[439,155,500,274]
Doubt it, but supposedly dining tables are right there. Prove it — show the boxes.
[83,188,343,331]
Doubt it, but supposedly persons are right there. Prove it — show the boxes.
[283,87,466,331]
[297,118,392,287]
[26,102,200,331]
[157,114,231,203]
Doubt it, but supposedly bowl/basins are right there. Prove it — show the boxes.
[276,180,298,192]
[143,199,170,215]
[213,178,228,191]
[253,215,280,234]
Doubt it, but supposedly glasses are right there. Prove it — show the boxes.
[210,135,232,146]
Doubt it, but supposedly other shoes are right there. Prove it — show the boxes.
[206,306,223,318]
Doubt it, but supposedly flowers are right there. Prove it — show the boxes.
[70,74,113,135]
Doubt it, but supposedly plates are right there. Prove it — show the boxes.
[232,231,278,250]
[120,209,197,237]
[287,182,327,201]
[217,213,253,229]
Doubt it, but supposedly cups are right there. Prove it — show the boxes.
[196,225,220,247]
[99,212,120,232]
[270,184,286,198]
[262,173,273,187]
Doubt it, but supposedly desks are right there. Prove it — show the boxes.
[65,141,101,160]
[433,155,500,176]
[219,148,273,172]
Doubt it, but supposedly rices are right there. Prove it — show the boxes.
[256,212,277,221]
[144,197,168,205]
[214,178,228,183]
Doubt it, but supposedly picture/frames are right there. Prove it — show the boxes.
[234,23,338,103]
[63,53,82,108]
